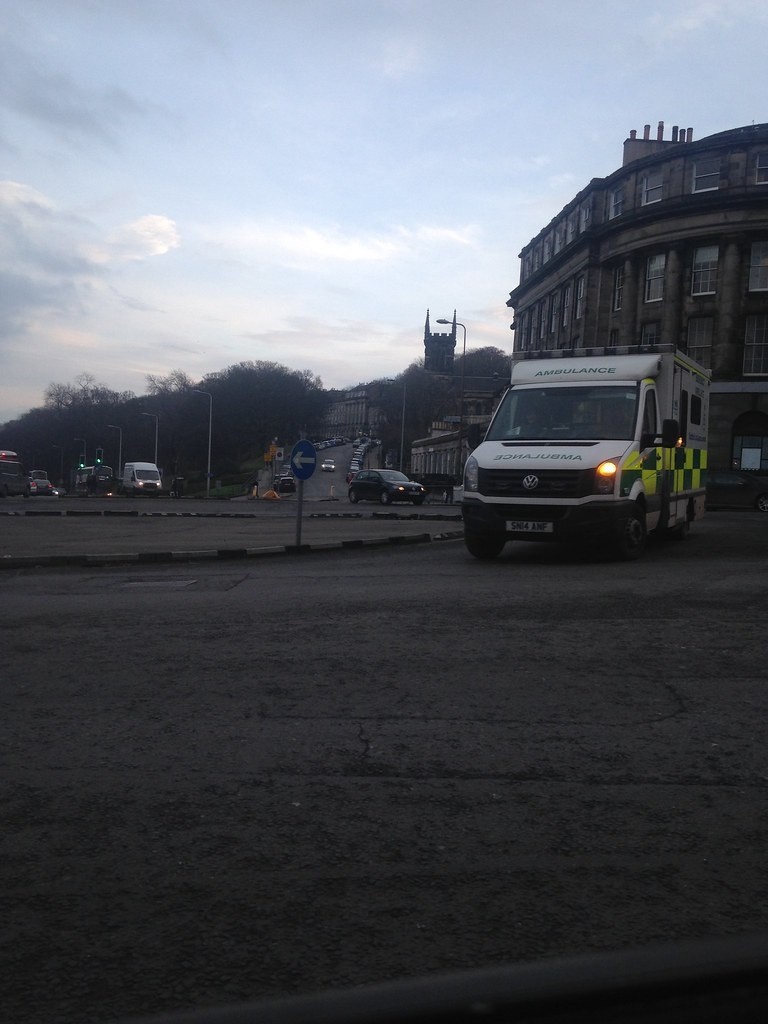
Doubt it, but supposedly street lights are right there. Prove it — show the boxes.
[436,318,466,488]
[52,445,64,489]
[387,379,406,473]
[74,438,87,469]
[192,389,213,498]
[140,412,159,467]
[108,425,122,479]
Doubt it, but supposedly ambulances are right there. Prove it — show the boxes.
[462,343,710,559]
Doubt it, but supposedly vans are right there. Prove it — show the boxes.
[123,462,162,498]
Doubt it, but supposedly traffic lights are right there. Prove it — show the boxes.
[95,449,103,464]
[78,454,86,468]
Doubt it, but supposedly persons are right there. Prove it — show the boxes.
[445,476,454,504]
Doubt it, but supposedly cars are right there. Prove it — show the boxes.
[349,470,425,505]
[706,471,768,513]
[0,451,52,498]
[321,459,337,473]
[274,438,381,492]
